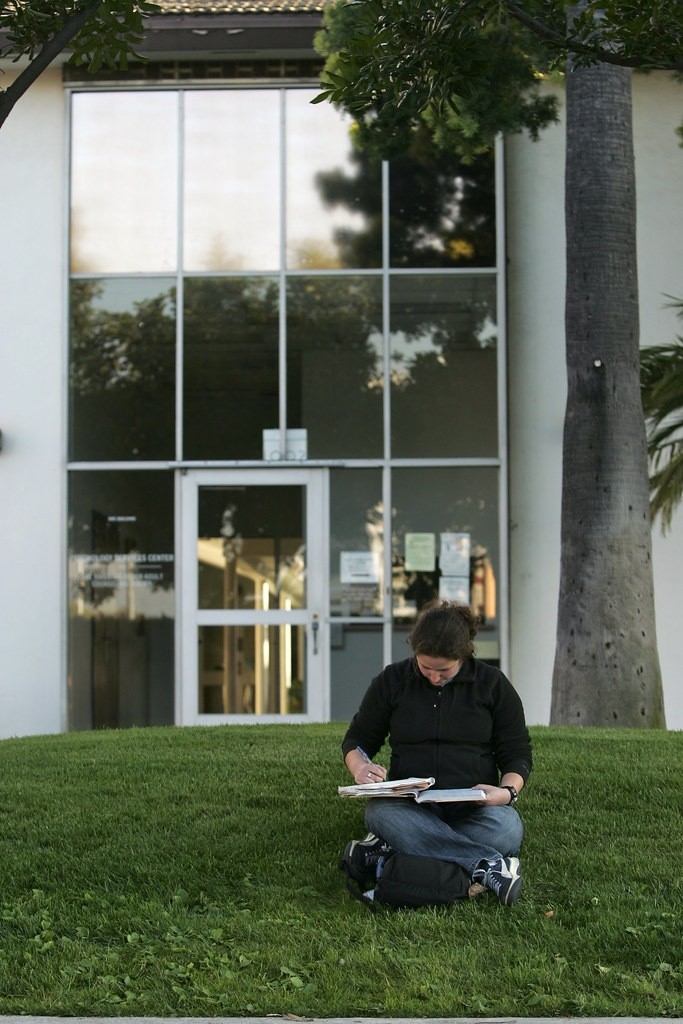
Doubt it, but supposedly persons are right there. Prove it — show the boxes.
[341,601,534,906]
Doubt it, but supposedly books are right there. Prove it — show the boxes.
[338,777,487,803]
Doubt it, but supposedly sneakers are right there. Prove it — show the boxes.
[343,831,393,880]
[473,856,525,908]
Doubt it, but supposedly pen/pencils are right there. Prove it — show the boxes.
[355,745,388,782]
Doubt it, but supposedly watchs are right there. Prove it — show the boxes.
[502,786,518,806]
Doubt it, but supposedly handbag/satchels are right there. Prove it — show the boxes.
[374,853,469,909]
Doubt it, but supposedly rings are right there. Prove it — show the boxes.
[368,773,371,777]
